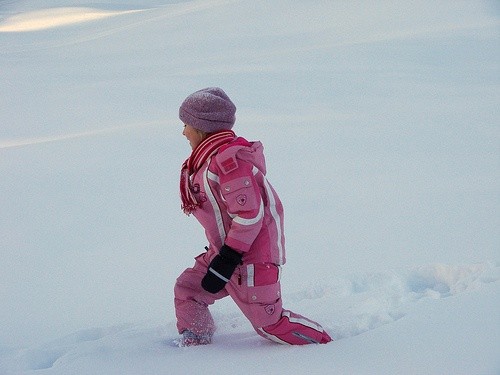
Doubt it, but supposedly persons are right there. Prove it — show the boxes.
[174,87,335,348]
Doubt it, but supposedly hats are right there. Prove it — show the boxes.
[179,87,236,133]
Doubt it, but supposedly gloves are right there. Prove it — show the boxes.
[201,243,245,294]
